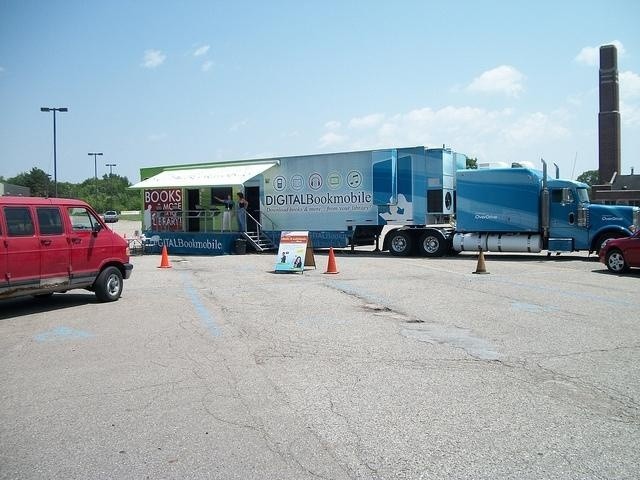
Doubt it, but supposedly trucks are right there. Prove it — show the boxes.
[382,158,640,257]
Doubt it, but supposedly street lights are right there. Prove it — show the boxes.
[86,152,104,196]
[105,162,116,174]
[41,105,68,197]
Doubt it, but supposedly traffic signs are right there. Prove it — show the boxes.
[156,244,174,268]
[323,247,340,275]
[472,248,491,274]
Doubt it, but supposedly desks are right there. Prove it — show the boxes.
[124,237,151,255]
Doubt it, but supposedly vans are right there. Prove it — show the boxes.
[0,196,134,302]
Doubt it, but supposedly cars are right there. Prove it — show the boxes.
[104,210,119,223]
[598,230,640,273]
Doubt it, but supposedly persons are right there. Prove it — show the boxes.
[214,194,235,232]
[237,193,248,232]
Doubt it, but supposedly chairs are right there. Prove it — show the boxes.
[142,235,159,255]
